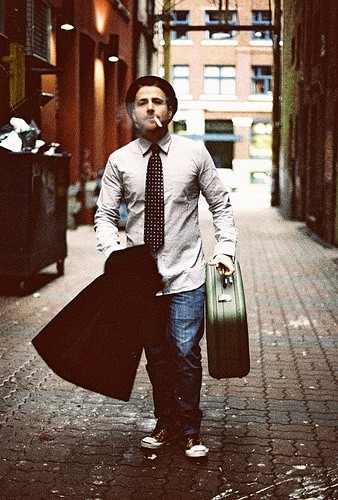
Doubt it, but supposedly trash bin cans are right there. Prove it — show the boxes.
[0,92,71,291]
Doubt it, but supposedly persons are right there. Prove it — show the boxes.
[80,147,91,180]
[95,170,104,195]
[94,77,237,457]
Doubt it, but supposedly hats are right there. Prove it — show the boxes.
[124,76,179,125]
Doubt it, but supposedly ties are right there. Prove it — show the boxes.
[145,145,164,253]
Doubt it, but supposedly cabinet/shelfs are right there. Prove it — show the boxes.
[1,153,72,293]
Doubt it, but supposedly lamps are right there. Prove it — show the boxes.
[98,33,120,63]
[55,0,77,34]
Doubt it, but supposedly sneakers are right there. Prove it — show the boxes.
[180,436,209,458]
[140,426,174,448]
